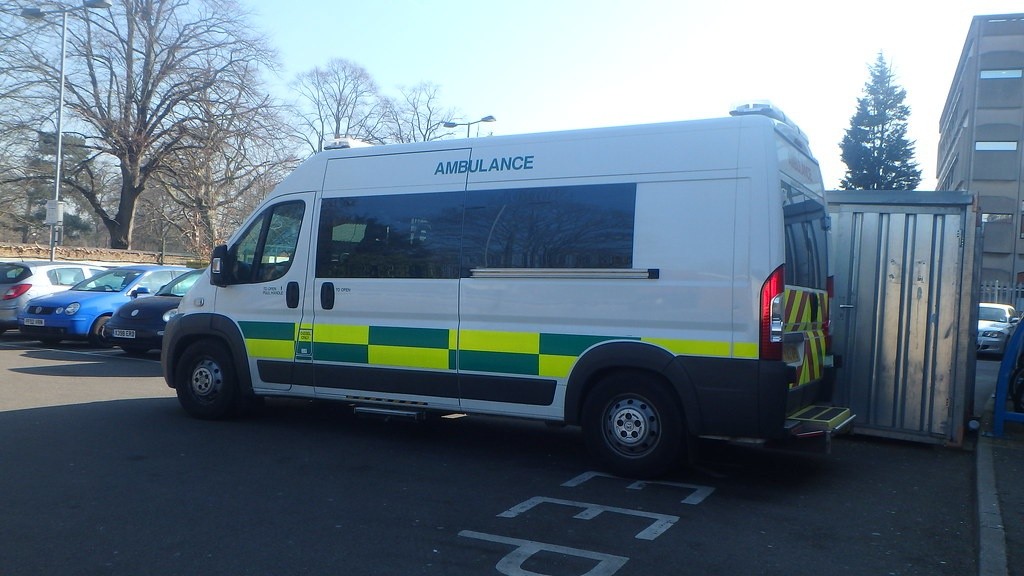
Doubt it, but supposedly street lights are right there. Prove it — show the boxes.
[20,0,116,262]
[444,116,498,139]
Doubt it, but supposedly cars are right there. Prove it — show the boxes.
[973,303,1020,360]
[17,266,199,351]
[101,267,205,358]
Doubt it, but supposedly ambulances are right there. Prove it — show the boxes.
[160,102,862,480]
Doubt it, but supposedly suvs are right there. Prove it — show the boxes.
[0,260,116,335]
